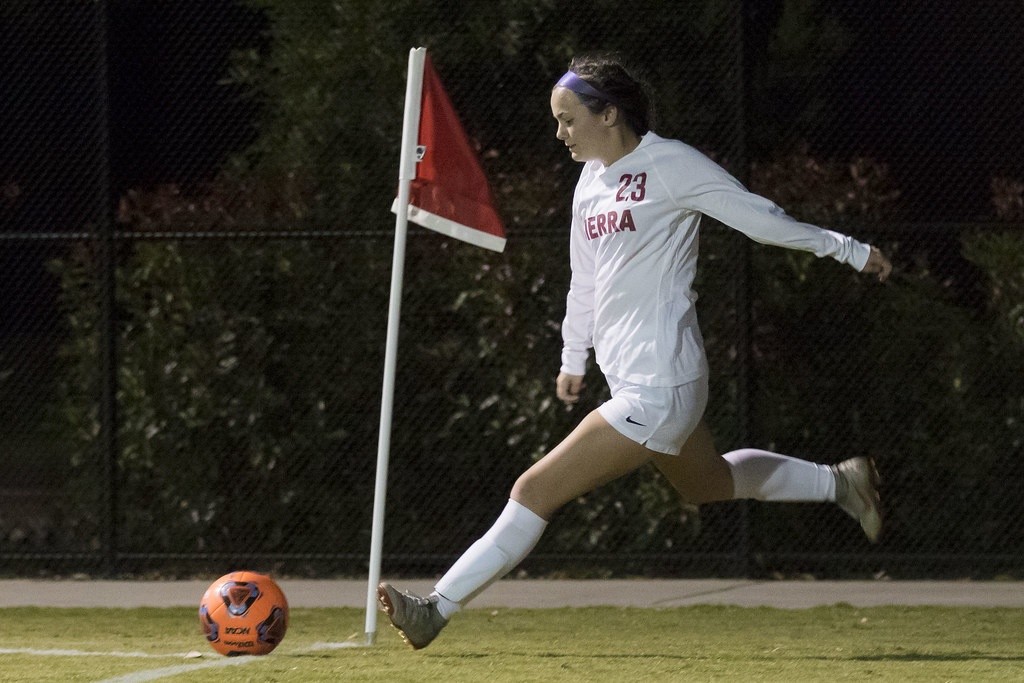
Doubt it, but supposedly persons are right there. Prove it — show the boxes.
[377,63,892,649]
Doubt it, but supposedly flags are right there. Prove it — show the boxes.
[390,52,507,253]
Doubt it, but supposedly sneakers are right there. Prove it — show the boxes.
[376,582,450,650]
[839,456,884,546]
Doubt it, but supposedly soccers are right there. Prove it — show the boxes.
[197,571,291,658]
